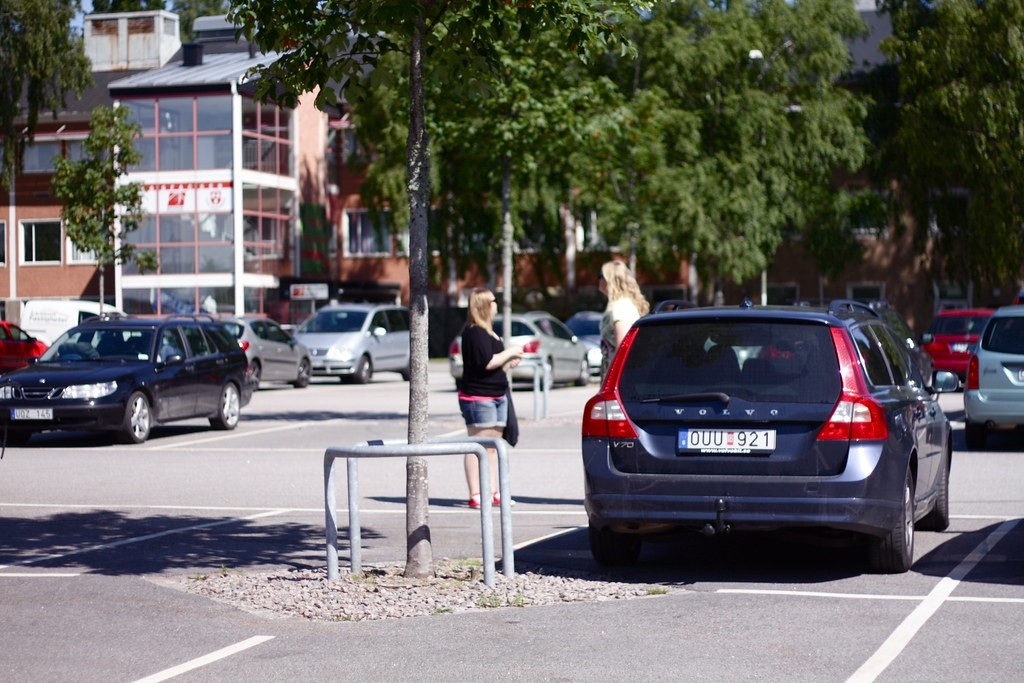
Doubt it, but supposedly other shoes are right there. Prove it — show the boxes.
[493,491,515,507]
[470,493,481,509]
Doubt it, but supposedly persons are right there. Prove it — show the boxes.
[599,260,650,389]
[458,287,524,509]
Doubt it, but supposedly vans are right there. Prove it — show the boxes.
[19,299,143,350]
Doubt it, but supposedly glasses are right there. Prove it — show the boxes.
[598,273,604,280]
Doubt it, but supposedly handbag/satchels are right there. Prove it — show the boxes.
[503,386,519,447]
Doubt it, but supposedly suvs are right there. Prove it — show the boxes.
[0,311,258,445]
[579,297,961,576]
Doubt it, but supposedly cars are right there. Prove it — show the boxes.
[292,302,410,384]
[218,314,313,388]
[447,310,590,389]
[962,305,1024,437]
[923,306,997,383]
[564,311,605,375]
[0,320,49,372]
